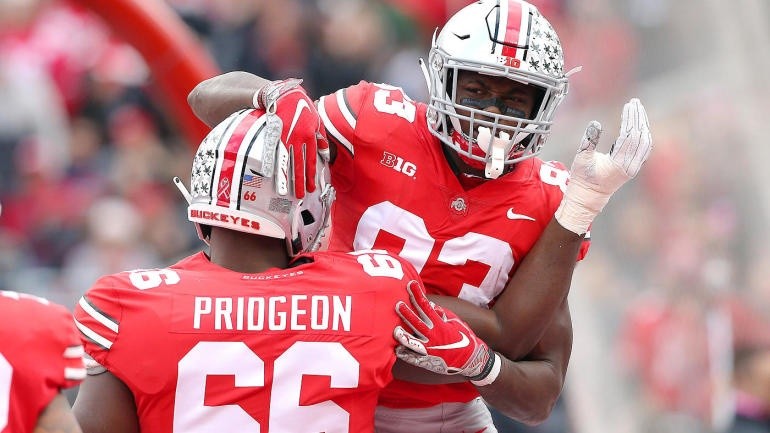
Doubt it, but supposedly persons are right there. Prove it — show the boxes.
[0,291,87,433]
[187,0,591,433]
[71,97,653,433]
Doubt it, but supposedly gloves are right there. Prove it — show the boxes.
[252,77,328,199]
[392,279,501,386]
[555,96,652,238]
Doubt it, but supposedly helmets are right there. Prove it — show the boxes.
[427,0,564,170]
[188,107,331,261]
